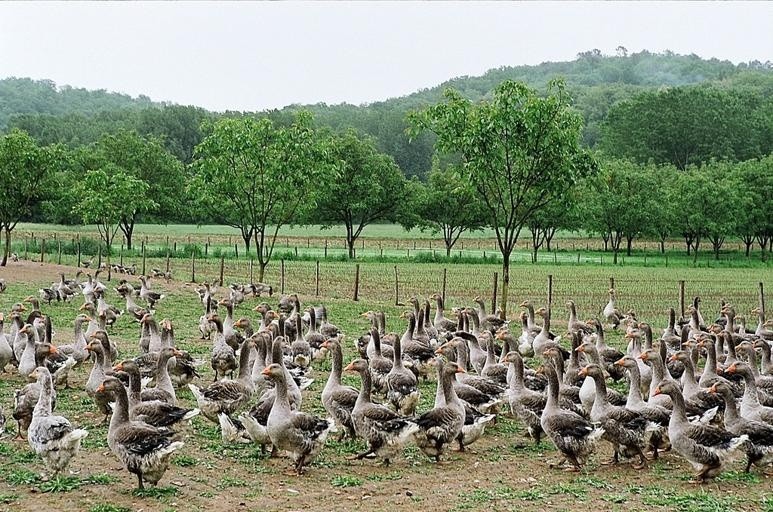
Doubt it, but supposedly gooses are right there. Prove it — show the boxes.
[0,251,773,499]
